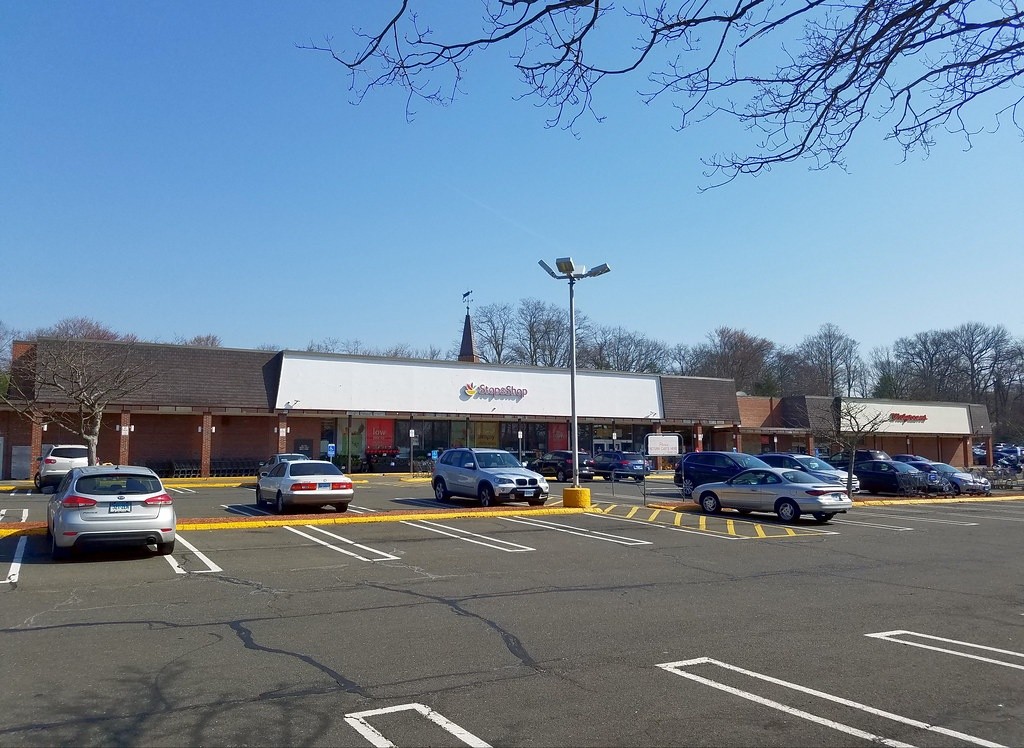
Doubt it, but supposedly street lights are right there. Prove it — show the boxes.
[538,255,611,488]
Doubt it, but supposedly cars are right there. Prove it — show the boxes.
[257,452,311,481]
[891,454,931,465]
[256,459,354,515]
[691,466,852,523]
[46,463,178,558]
[839,459,946,498]
[972,441,1024,469]
[904,460,992,497]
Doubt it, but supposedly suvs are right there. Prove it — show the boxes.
[592,450,650,483]
[33,443,102,490]
[430,447,550,508]
[753,452,860,495]
[819,449,893,470]
[674,451,774,500]
[529,449,597,482]
